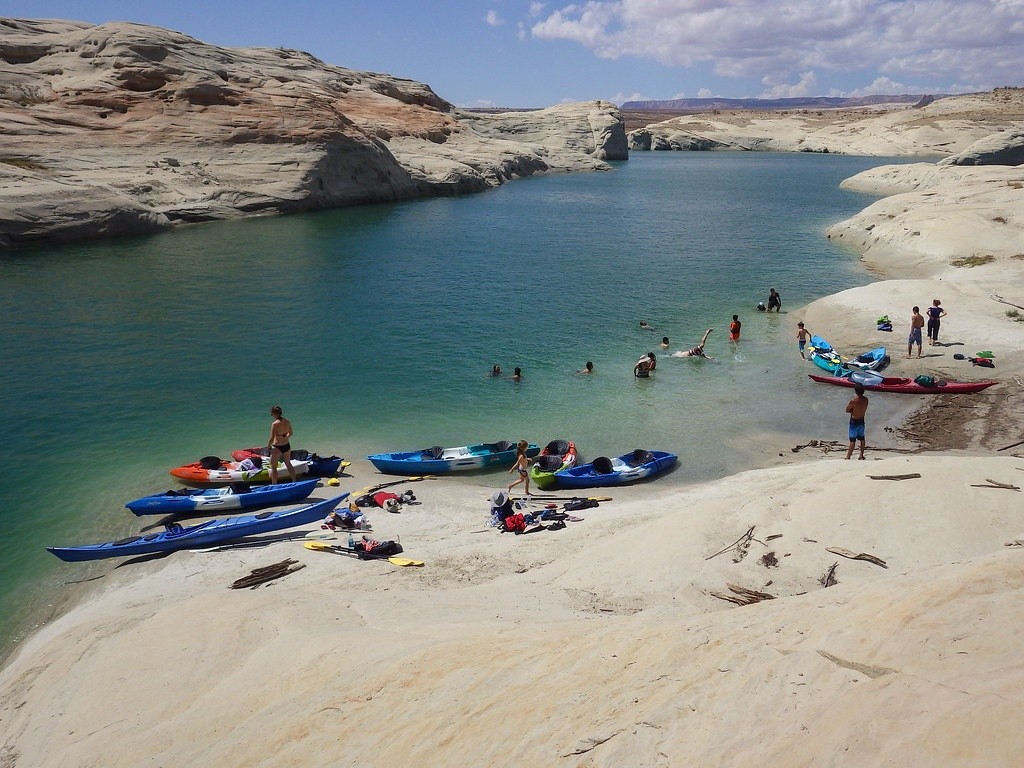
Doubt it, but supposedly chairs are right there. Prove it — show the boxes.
[198,456,228,472]
[421,445,444,460]
[628,448,654,467]
[242,456,262,470]
[858,355,874,363]
[290,449,308,461]
[538,456,562,472]
[591,456,612,475]
[816,349,830,354]
[496,440,512,451]
[544,439,569,456]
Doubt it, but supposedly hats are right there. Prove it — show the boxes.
[490,490,509,508]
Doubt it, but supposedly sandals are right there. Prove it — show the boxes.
[547,520,565,530]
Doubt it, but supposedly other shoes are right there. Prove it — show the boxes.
[328,524,336,531]
[321,525,327,530]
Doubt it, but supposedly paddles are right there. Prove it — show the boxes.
[807,346,840,364]
[304,541,424,567]
[352,477,425,497]
[487,495,613,501]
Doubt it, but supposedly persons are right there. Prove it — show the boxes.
[513,367,521,378]
[582,361,593,373]
[768,288,781,312]
[634,354,650,378]
[756,301,767,312]
[927,299,947,345]
[493,364,500,372]
[681,328,713,357]
[731,314,742,338]
[489,489,515,521]
[648,352,656,370]
[266,404,296,485]
[795,321,811,358]
[508,440,534,496]
[640,320,647,328]
[661,337,669,348]
[844,381,869,459]
[906,306,924,359]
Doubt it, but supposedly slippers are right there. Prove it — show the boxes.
[566,515,583,522]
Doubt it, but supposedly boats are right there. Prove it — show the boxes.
[554,448,678,489]
[366,440,540,475]
[45,492,351,562]
[231,446,345,476]
[124,478,321,518]
[531,438,578,489]
[807,335,843,373]
[834,344,887,379]
[807,372,1000,394]
[170,456,313,486]
